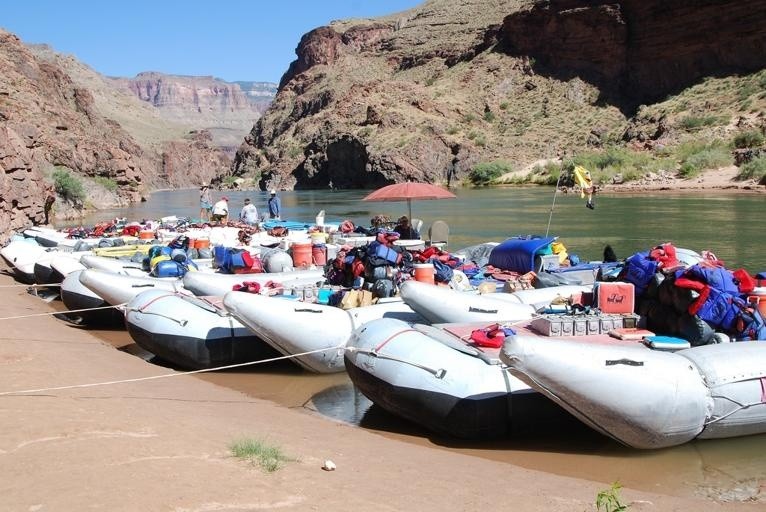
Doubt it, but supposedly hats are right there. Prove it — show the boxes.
[270,190,276,194]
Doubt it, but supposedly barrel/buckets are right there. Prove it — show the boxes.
[413,263,435,284]
[312,247,326,267]
[194,240,210,249]
[138,230,155,239]
[289,244,313,268]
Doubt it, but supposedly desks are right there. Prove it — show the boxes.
[393,239,425,251]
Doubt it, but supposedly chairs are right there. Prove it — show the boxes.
[410,218,449,253]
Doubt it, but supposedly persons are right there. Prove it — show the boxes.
[199,182,213,222]
[212,195,229,221]
[268,189,280,219]
[240,198,258,224]
[393,216,420,239]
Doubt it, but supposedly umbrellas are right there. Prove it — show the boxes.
[362,180,456,238]
